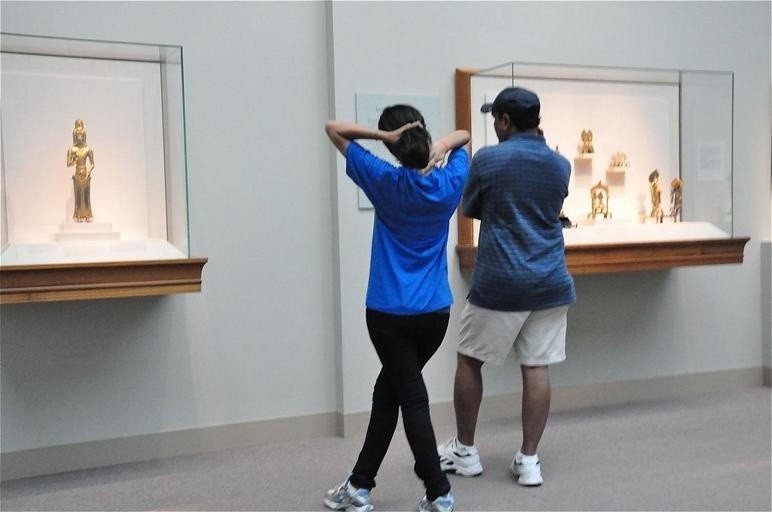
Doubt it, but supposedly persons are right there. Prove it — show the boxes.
[322,102,472,512]
[435,84,574,487]
[65,119,97,225]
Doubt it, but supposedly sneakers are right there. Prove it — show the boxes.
[436,434,483,477]
[418,489,454,512]
[324,474,374,512]
[510,449,544,487]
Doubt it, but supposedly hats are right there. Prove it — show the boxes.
[480,87,541,132]
